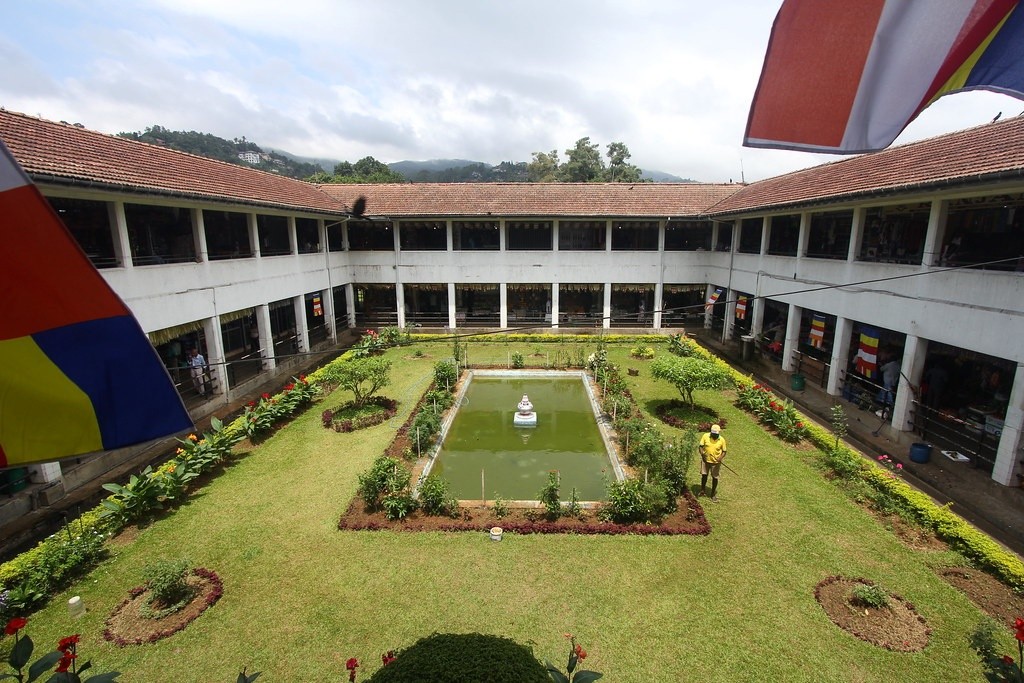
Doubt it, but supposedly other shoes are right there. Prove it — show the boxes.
[710,496,719,503]
[698,490,705,495]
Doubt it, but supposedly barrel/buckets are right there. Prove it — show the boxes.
[910,441,932,463]
[790,373,805,391]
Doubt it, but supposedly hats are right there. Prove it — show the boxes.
[711,424,721,434]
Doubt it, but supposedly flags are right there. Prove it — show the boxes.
[740,0,1023,155]
[855,325,880,380]
[1,139,194,468]
[807,310,826,350]
[735,292,749,320]
[312,291,323,316]
[704,287,724,310]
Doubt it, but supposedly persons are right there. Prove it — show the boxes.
[188,349,207,396]
[877,355,900,405]
[698,424,727,502]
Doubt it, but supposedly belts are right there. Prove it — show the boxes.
[191,367,200,370]
